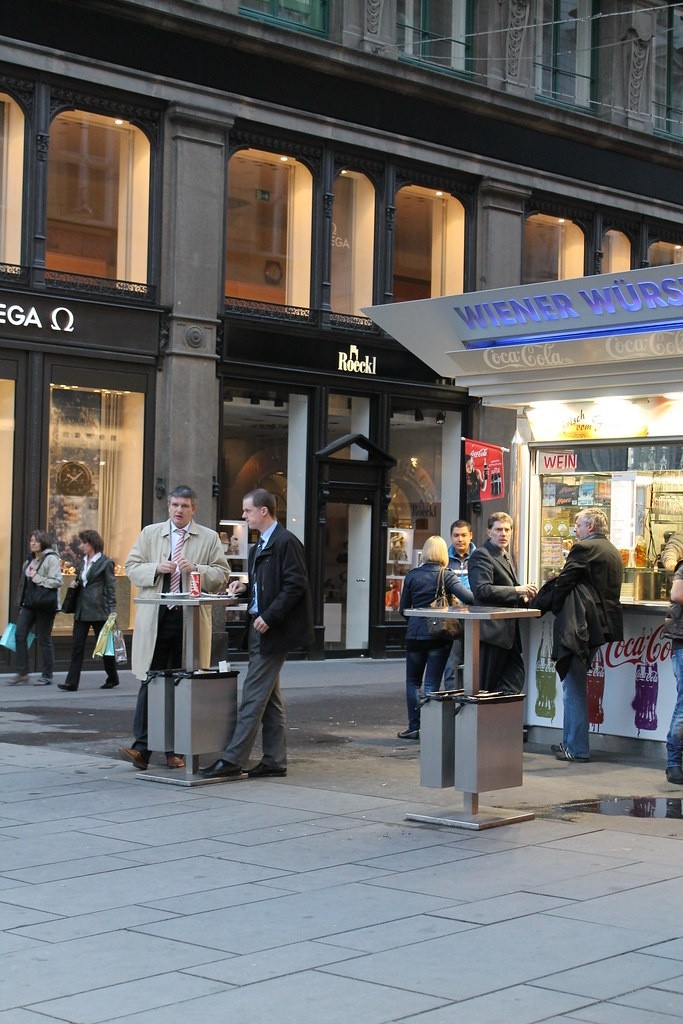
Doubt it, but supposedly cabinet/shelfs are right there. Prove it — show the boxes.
[216,518,252,650]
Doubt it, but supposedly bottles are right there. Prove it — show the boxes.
[634,627,660,730]
[580,640,606,723]
[535,618,555,719]
[484,458,502,495]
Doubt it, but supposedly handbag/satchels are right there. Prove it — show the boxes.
[0,623,36,654]
[91,613,127,665]
[428,566,463,639]
[22,578,57,613]
[60,587,79,613]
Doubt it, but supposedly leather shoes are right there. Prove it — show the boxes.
[118,746,148,770]
[248,762,287,777]
[197,760,242,778]
[166,754,183,768]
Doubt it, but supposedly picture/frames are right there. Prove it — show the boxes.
[384,576,406,612]
[386,528,413,564]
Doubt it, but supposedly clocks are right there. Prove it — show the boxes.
[56,461,94,497]
[264,261,283,285]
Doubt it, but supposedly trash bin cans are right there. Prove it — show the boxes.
[145,668,186,752]
[418,689,487,790]
[451,692,527,792]
[171,668,240,755]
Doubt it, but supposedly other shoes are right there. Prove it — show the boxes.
[33,677,53,686]
[665,765,683,784]
[4,675,29,685]
[100,681,119,689]
[57,683,77,691]
[398,729,419,740]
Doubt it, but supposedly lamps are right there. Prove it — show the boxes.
[251,398,260,404]
[436,411,447,423]
[274,400,283,407]
[224,392,233,401]
[415,409,424,420]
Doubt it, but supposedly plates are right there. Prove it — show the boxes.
[156,592,238,597]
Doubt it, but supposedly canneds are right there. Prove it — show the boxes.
[190,573,200,598]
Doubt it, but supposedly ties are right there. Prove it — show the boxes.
[246,538,265,610]
[166,529,185,610]
[504,553,512,570]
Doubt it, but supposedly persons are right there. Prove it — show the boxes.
[57,530,120,691]
[465,455,489,503]
[444,520,478,690]
[197,488,317,778]
[660,529,683,571]
[120,485,232,770]
[467,511,539,743]
[397,535,474,739]
[662,560,683,784]
[529,507,624,763]
[5,530,64,686]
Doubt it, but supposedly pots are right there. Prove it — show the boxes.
[615,566,673,600]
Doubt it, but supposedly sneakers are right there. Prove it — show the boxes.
[551,743,566,753]
[555,750,588,762]
